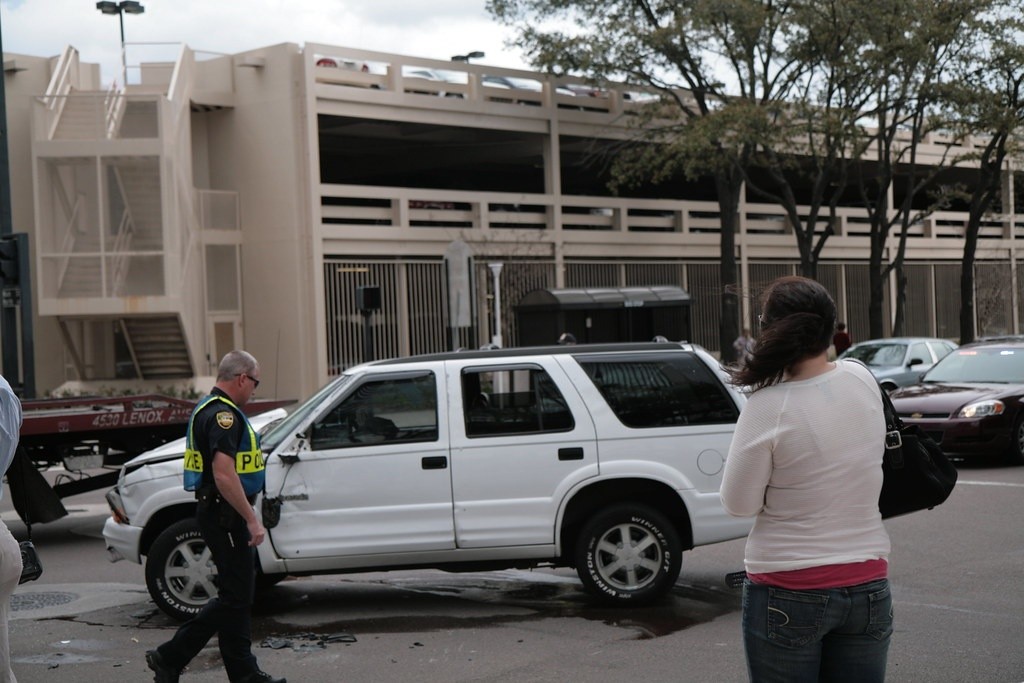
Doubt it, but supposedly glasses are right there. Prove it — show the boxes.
[233,373,260,389]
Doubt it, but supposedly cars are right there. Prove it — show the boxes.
[836,337,959,396]
[893,339,1024,467]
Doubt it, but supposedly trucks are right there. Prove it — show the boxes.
[6,394,298,525]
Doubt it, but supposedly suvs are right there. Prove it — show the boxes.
[102,341,755,620]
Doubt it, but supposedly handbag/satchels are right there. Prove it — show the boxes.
[880,390,959,522]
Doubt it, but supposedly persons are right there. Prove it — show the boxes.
[832,323,850,356]
[720,278,894,683]
[0,374,22,683]
[540,334,575,418]
[733,328,756,366]
[144,349,288,683]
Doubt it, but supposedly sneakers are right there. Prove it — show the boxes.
[144,649,180,683]
[229,670,287,683]
